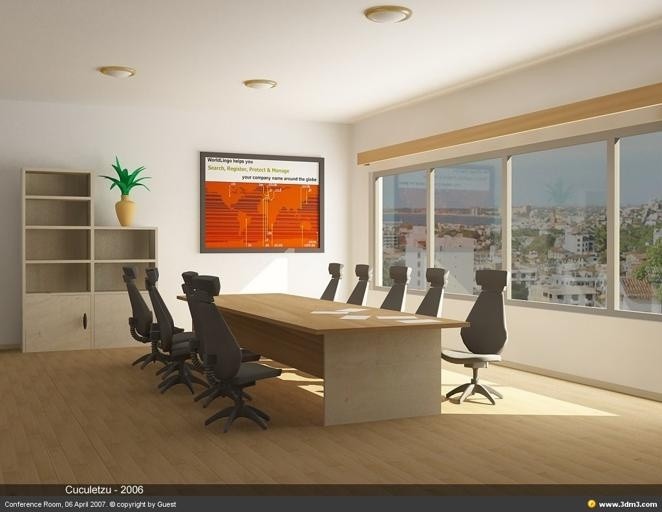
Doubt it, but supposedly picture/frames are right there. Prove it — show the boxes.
[198,152,326,254]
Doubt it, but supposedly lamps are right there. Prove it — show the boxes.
[363,5,413,24]
[243,79,276,90]
[99,65,136,79]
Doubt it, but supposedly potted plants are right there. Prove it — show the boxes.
[99,155,152,227]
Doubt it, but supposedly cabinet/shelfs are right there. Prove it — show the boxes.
[19,167,159,353]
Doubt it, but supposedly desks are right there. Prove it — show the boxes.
[174,291,472,428]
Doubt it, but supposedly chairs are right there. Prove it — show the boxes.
[120,263,281,433]
[319,261,511,406]
